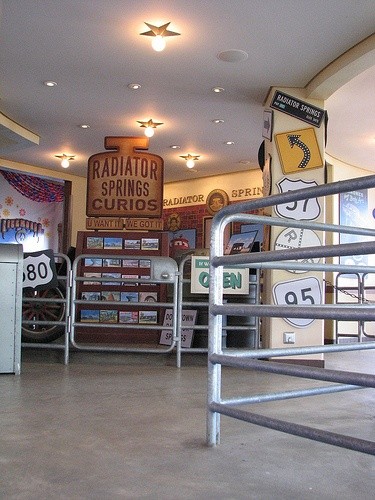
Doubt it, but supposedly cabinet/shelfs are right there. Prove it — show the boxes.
[75,230,168,340]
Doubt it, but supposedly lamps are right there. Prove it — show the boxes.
[137,119,164,138]
[181,154,200,168]
[0,113,40,155]
[140,21,181,51]
[56,154,75,168]
